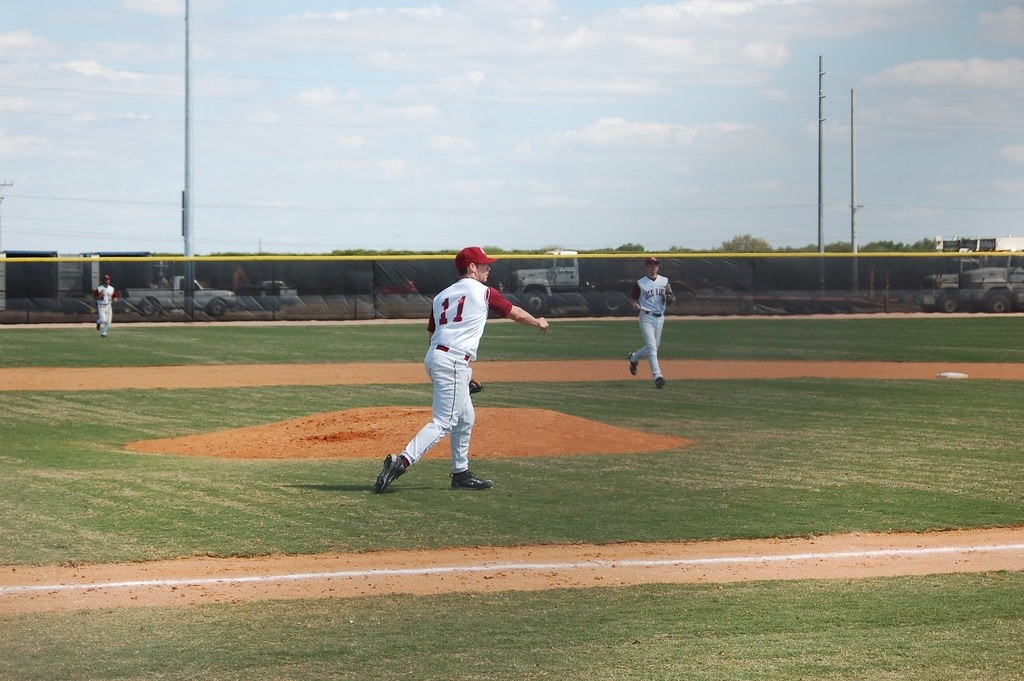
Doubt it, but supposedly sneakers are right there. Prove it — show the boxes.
[450,470,493,490]
[374,453,408,494]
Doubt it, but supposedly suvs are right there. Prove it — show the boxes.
[376,278,417,301]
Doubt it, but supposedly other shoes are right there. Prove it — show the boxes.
[101,330,107,337]
[655,377,665,388]
[627,352,638,375]
[97,322,100,331]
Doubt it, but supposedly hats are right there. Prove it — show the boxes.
[455,247,496,269]
[104,275,111,280]
[646,257,659,265]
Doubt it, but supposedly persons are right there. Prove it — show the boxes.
[627,257,676,389]
[94,275,118,337]
[147,271,169,290]
[375,247,550,494]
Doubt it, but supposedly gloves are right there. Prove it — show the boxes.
[667,294,676,305]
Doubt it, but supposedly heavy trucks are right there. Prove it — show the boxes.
[958,250,1024,312]
[921,255,979,313]
[506,251,853,316]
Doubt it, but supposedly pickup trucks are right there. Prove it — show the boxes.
[257,280,297,305]
[115,275,235,317]
